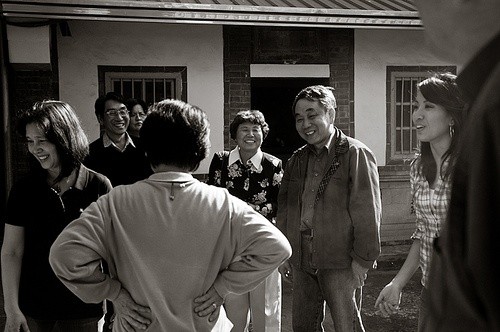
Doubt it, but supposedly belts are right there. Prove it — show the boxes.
[301,229,313,240]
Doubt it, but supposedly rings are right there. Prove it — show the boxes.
[212,303,217,309]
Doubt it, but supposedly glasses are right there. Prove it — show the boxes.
[104,109,130,117]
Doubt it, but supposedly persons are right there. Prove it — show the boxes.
[207,110,285,332]
[414,0,500,332]
[50,99,293,332]
[275,85,382,332]
[0,100,114,332]
[375,72,462,332]
[80,91,153,188]
